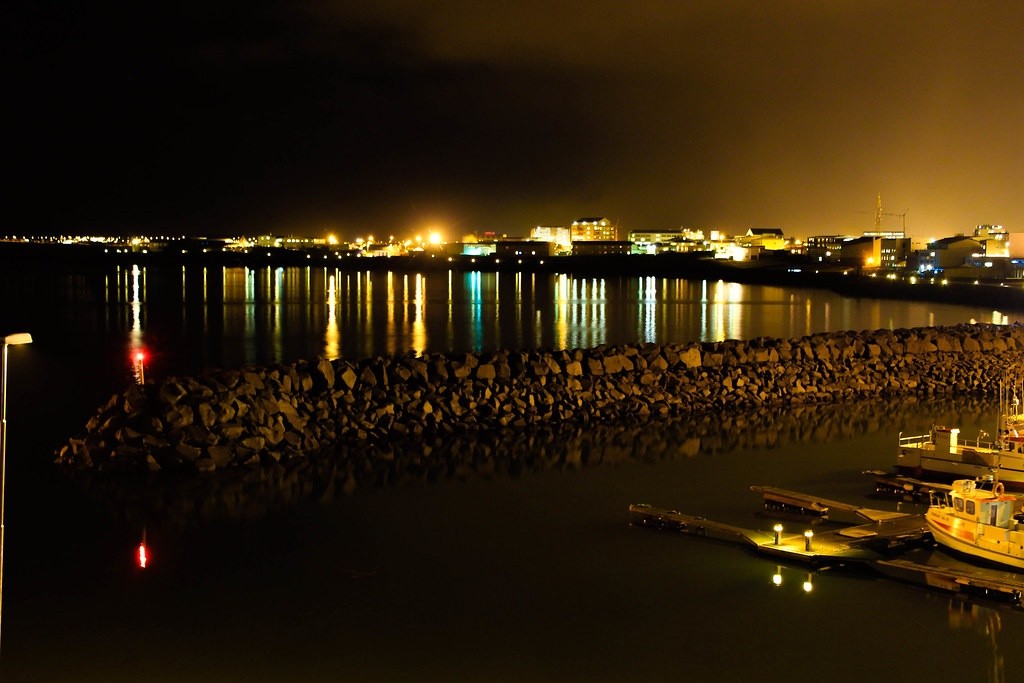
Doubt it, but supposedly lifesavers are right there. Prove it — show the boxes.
[992,482,1005,498]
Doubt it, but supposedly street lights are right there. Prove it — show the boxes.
[2,332,34,600]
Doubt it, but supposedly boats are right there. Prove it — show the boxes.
[924,477,1023,571]
[898,367,1024,493]
